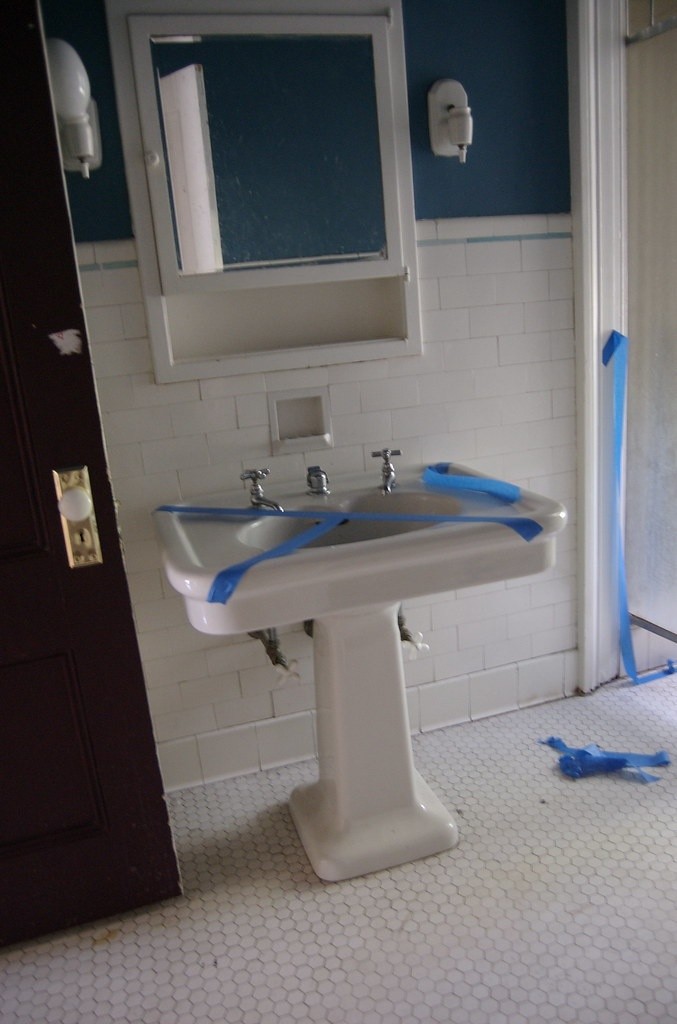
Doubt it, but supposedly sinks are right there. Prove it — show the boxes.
[150,460,568,636]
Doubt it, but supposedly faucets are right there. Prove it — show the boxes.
[371,449,403,498]
[239,468,285,514]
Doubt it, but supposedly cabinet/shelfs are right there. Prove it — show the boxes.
[107,0,424,385]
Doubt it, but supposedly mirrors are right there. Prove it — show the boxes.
[133,19,404,295]
